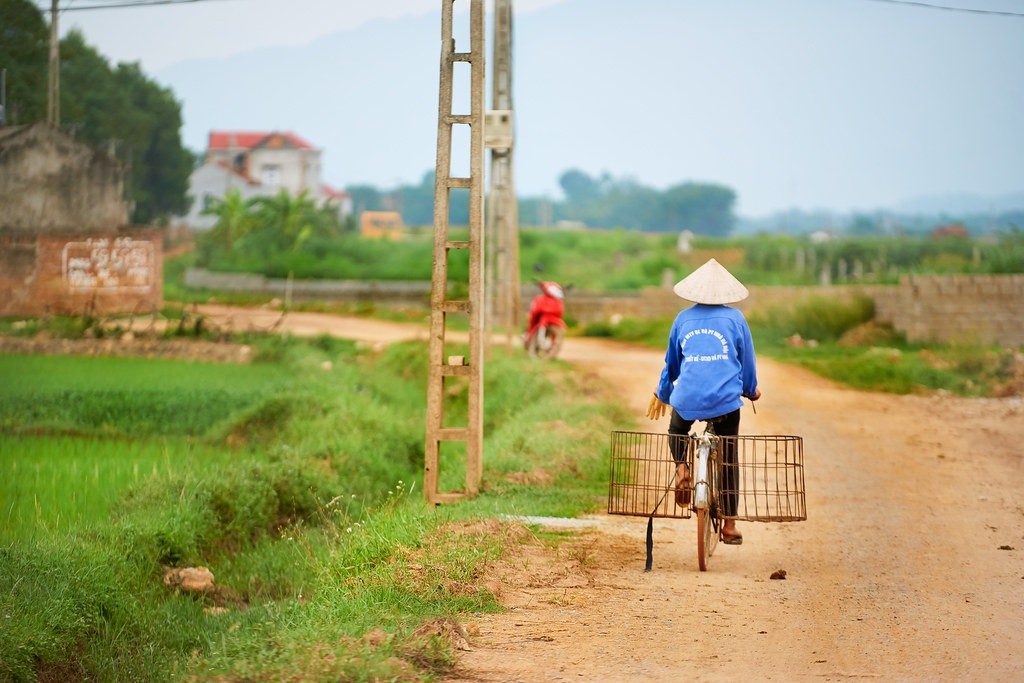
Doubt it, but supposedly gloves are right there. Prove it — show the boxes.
[645,394,666,420]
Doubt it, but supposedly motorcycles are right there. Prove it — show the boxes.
[525,263,575,362]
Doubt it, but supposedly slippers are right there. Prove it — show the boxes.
[724,533,741,543]
[675,477,692,508]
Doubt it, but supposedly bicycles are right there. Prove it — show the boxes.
[678,389,760,573]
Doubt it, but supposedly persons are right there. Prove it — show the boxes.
[641,257,763,537]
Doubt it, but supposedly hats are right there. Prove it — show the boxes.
[673,257,749,305]
